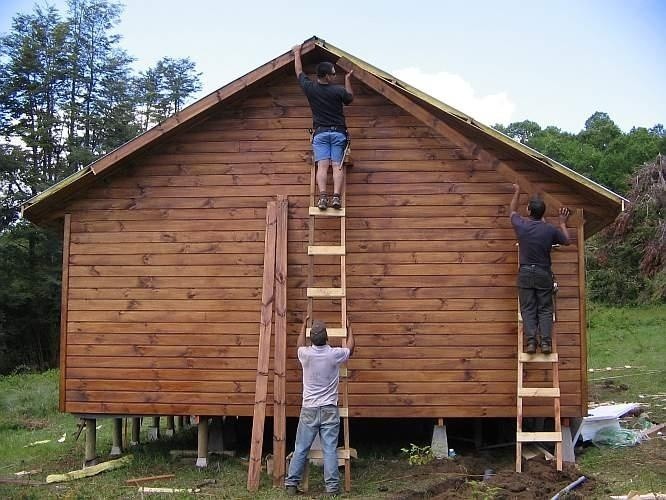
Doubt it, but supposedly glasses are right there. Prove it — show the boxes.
[328,72,336,76]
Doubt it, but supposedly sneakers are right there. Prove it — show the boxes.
[541,343,552,353]
[317,196,328,209]
[527,343,537,353]
[331,196,341,208]
[328,489,340,496]
[286,487,297,496]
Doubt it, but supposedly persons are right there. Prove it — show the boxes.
[508,177,572,354]
[283,313,355,496]
[291,43,354,210]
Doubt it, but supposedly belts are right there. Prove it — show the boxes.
[315,126,346,133]
[324,401,339,406]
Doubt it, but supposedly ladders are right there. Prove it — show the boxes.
[516,242,563,474]
[302,153,351,492]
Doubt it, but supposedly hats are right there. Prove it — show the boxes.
[310,319,327,345]
